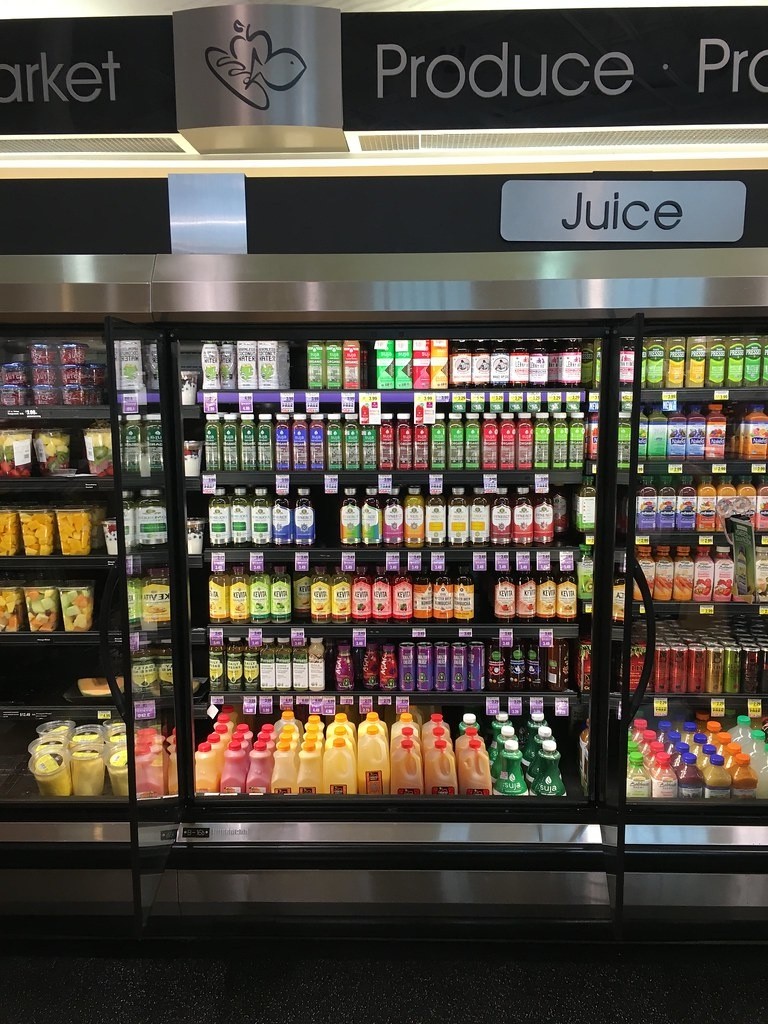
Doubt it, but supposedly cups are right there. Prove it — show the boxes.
[184,517,204,555]
[183,441,203,475]
[101,520,117,556]
[29,720,127,798]
[182,371,198,405]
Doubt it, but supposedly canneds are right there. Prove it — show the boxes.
[399,641,486,692]
[449,338,602,387]
[639,615,768,695]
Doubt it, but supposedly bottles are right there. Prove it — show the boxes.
[118,405,768,470]
[134,701,768,798]
[115,475,768,544]
[577,545,594,598]
[133,638,398,693]
[633,547,768,602]
[128,567,597,623]
